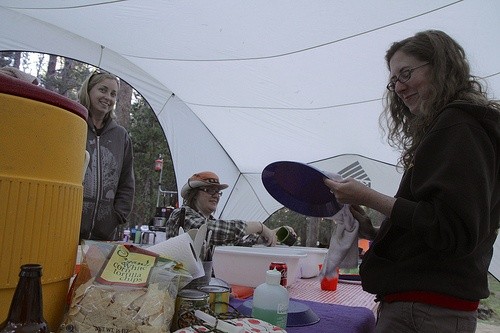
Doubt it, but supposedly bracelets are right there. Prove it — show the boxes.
[257,221,263,234]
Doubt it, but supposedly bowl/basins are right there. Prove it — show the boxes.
[211,243,329,288]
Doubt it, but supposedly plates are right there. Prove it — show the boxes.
[237,300,321,326]
[262,161,344,218]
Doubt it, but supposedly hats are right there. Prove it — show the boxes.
[181,171,229,199]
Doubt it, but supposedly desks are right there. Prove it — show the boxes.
[229,289,380,333]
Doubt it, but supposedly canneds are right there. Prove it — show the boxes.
[269,262,288,288]
[171,284,230,333]
[141,231,156,245]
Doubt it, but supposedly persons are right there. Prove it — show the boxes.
[79,70,135,246]
[166,171,278,288]
[322,29,500,333]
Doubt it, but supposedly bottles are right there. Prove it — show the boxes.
[0,263,50,333]
[252,270,289,330]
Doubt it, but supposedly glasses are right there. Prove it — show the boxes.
[89,69,119,83]
[199,188,222,197]
[387,63,431,92]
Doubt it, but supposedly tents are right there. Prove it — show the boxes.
[0,0,499,282]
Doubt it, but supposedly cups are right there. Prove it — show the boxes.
[317,263,339,291]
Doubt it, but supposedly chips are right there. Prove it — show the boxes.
[55,276,175,333]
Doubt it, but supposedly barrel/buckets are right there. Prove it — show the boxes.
[0,61,91,333]
